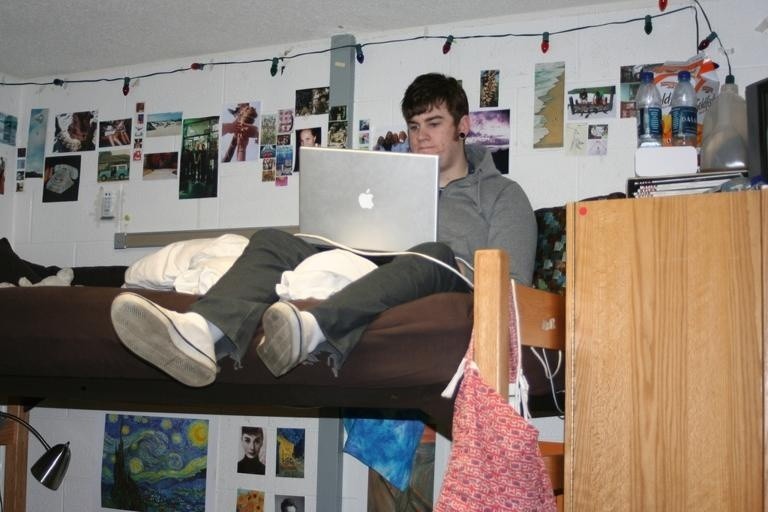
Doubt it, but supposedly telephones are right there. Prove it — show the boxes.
[95,187,115,219]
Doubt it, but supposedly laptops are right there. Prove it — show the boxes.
[298,146,440,252]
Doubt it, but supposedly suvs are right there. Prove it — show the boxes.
[98,161,129,182]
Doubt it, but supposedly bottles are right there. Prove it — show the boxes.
[635,71,662,146]
[721,175,762,192]
[700,84,750,170]
[671,70,698,146]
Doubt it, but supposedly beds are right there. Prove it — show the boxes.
[1,238,564,511]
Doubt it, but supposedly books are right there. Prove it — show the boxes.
[627,173,747,199]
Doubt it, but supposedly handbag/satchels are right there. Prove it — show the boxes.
[436,361,555,512]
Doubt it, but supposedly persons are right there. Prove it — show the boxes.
[237,426,265,474]
[297,129,322,149]
[102,122,123,146]
[280,499,298,512]
[112,120,131,145]
[110,73,539,389]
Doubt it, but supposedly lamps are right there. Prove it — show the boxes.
[0,408,73,491]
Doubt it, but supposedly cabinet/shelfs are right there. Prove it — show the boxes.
[563,174,768,512]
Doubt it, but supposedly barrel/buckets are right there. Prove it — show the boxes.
[699,74,748,173]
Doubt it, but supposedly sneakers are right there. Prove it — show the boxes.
[255,300,305,378]
[110,292,218,388]
